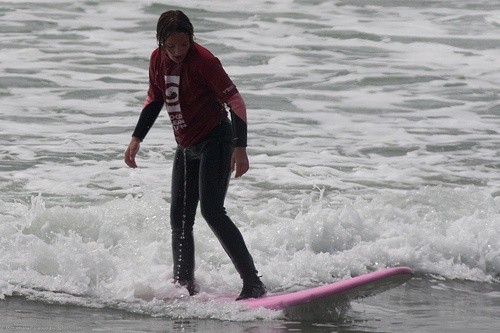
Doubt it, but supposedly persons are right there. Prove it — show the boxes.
[126,10,268,301]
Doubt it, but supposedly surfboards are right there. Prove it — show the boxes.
[214,267,413,310]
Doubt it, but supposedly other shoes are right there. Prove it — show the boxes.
[235,280,266,301]
